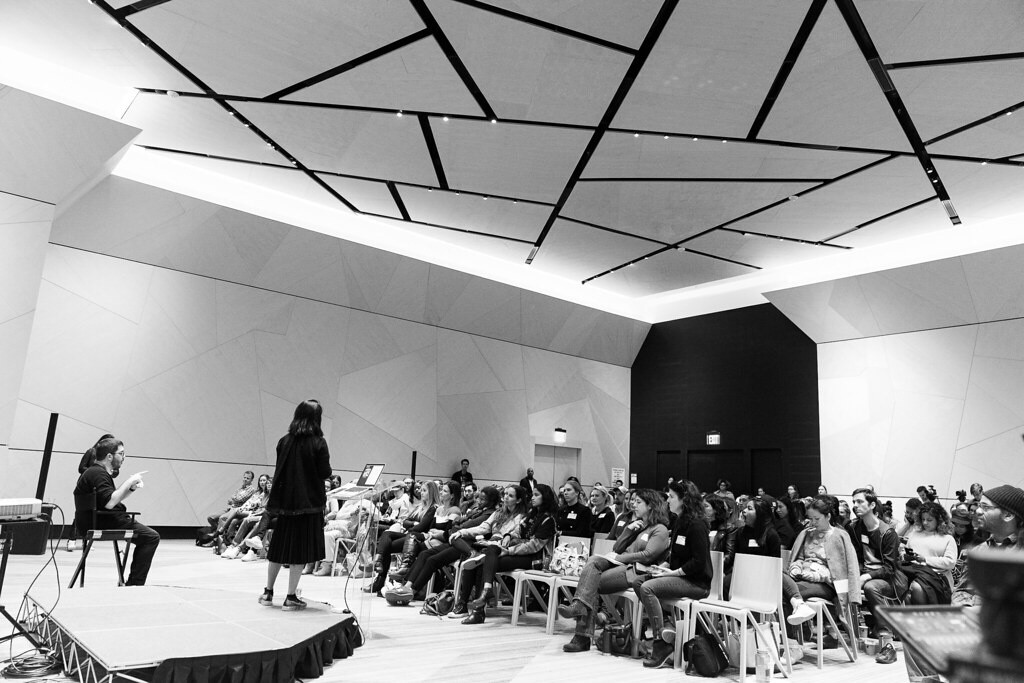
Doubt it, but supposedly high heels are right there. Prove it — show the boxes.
[462,608,485,624]
[557,600,589,626]
[472,588,495,607]
[563,634,591,652]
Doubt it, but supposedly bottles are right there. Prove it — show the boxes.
[755,649,771,683]
[543,555,551,571]
[295,583,302,601]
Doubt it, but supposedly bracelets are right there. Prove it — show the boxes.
[129,488,136,492]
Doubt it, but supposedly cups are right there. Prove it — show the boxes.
[475,535,484,542]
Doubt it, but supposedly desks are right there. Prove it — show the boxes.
[0,516,49,602]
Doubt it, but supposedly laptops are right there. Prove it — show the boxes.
[333,464,385,497]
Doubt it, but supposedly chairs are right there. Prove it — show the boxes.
[378,529,857,683]
[66,487,140,586]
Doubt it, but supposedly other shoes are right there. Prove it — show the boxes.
[389,565,411,579]
[258,593,272,605]
[661,623,676,645]
[643,640,675,668]
[811,634,838,649]
[314,565,331,575]
[876,643,897,663]
[202,531,241,559]
[245,536,263,550]
[302,563,313,574]
[386,587,414,606]
[282,598,307,610]
[241,550,257,562]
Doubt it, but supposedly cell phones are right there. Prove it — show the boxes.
[905,548,914,556]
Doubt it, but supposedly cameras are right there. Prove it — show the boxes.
[956,490,966,496]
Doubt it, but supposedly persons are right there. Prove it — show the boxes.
[258,399,333,611]
[73,441,162,585]
[67,434,119,552]
[558,477,1024,683]
[202,457,557,624]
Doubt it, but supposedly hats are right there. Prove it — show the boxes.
[950,509,973,525]
[983,485,1024,521]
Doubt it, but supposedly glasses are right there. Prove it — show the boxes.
[112,451,125,457]
[977,502,1000,511]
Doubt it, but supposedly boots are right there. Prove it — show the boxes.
[359,554,383,573]
[361,585,372,592]
[787,597,816,625]
[779,637,803,666]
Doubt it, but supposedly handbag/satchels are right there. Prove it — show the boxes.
[595,622,633,655]
[421,590,455,616]
[549,541,589,577]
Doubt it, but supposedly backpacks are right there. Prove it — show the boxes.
[683,632,729,677]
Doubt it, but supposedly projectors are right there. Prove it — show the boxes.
[0,498,42,521]
